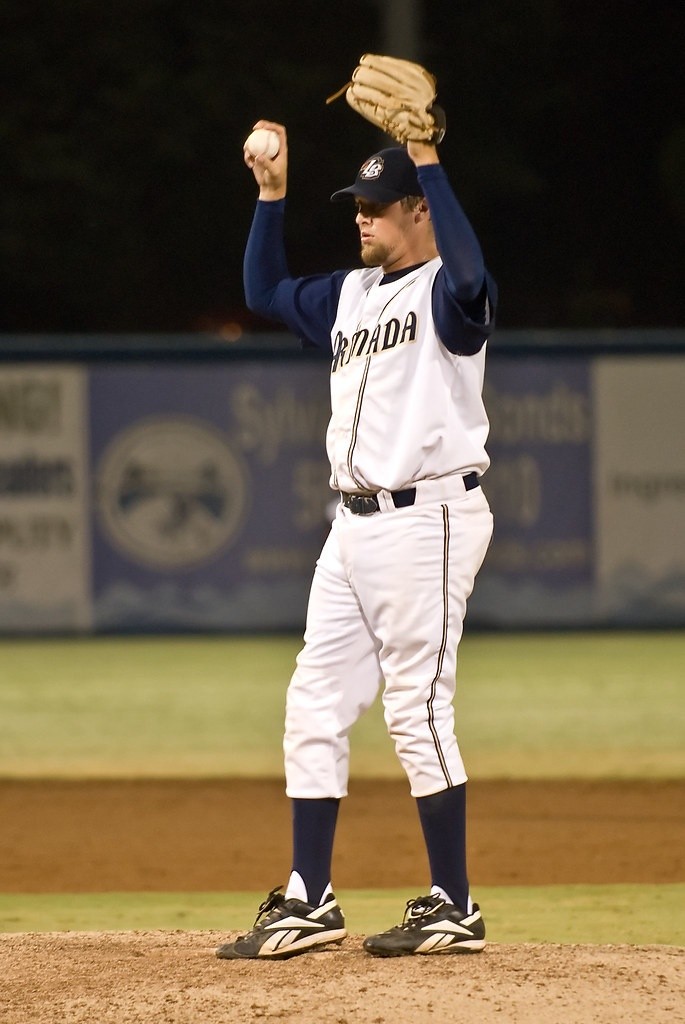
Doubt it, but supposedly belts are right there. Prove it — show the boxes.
[343,472,481,515]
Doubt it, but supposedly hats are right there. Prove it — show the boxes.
[329,147,424,204]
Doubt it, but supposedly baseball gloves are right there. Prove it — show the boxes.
[346,53,447,145]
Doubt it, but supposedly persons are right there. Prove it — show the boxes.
[215,53,501,959]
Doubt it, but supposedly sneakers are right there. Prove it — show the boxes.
[216,870,347,959]
[362,885,486,958]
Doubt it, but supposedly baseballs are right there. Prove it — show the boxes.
[247,129,280,159]
